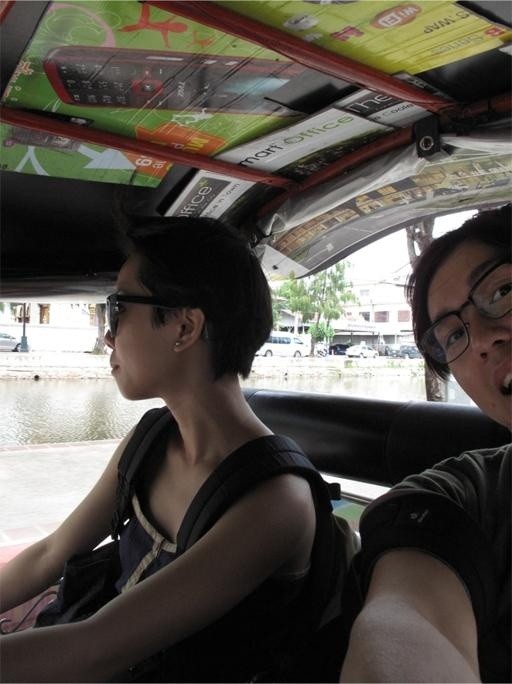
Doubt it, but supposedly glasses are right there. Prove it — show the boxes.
[105,293,182,337]
[418,259,512,365]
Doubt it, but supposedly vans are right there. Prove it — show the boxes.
[254,332,309,358]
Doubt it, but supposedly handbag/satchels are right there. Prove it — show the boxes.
[31,539,124,628]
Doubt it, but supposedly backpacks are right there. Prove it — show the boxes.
[110,406,358,683]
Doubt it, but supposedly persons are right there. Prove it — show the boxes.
[336,201,512,683]
[0,214,318,683]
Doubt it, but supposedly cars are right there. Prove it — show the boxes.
[0,330,22,352]
[328,340,425,359]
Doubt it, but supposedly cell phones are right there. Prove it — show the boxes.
[44,46,371,116]
